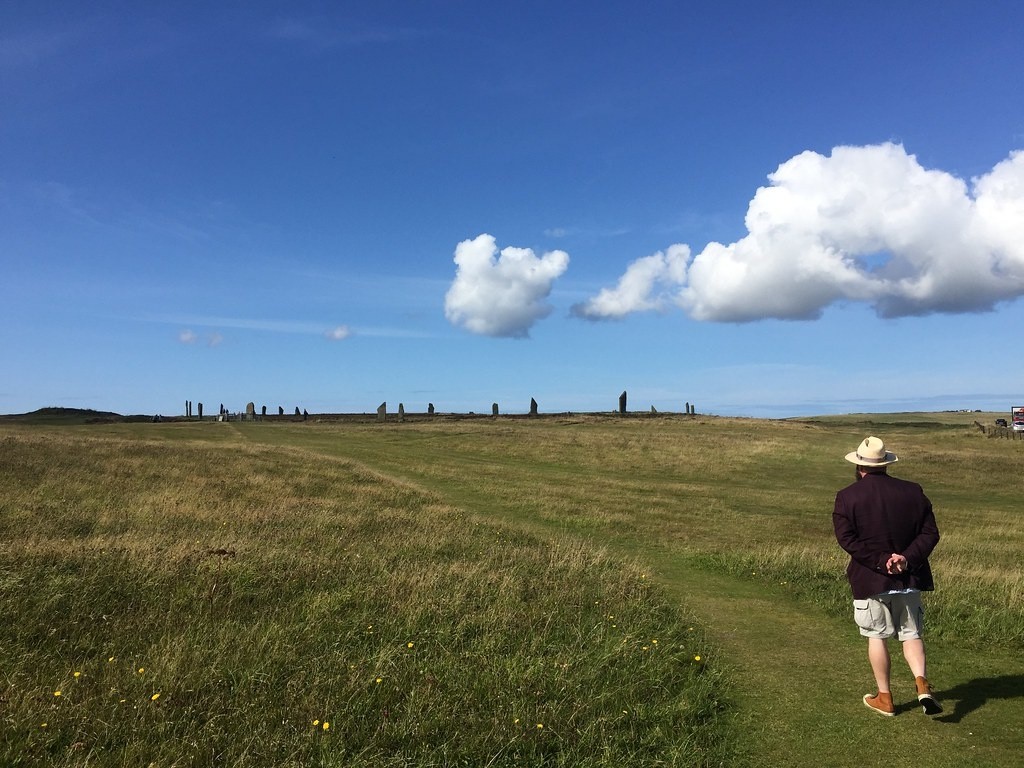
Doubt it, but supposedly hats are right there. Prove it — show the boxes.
[845,436,898,467]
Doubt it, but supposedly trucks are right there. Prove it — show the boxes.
[1011,406,1024,421]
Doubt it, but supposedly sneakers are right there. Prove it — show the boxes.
[915,676,943,715]
[863,691,896,716]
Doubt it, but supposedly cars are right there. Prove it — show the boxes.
[995,419,1008,427]
[1011,420,1024,431]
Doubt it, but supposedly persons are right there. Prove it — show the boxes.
[832,437,940,717]
